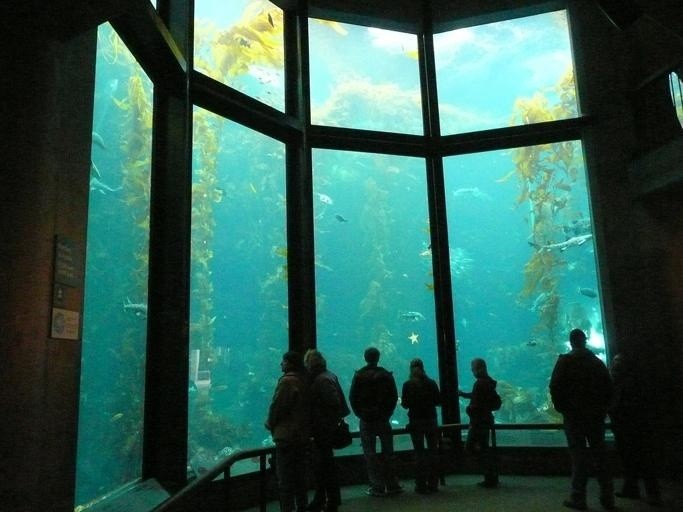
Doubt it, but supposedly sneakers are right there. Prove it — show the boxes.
[388,481,407,496]
[415,480,426,492]
[429,478,440,491]
[365,483,384,497]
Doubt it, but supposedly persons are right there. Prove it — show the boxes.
[455,359,497,489]
[348,349,403,496]
[265,351,315,512]
[607,352,661,506]
[549,329,617,512]
[401,360,442,493]
[302,348,350,512]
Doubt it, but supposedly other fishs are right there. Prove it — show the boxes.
[524,231,596,256]
[393,307,431,329]
[577,287,600,301]
[122,297,149,317]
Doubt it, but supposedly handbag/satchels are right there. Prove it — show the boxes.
[334,419,352,449]
